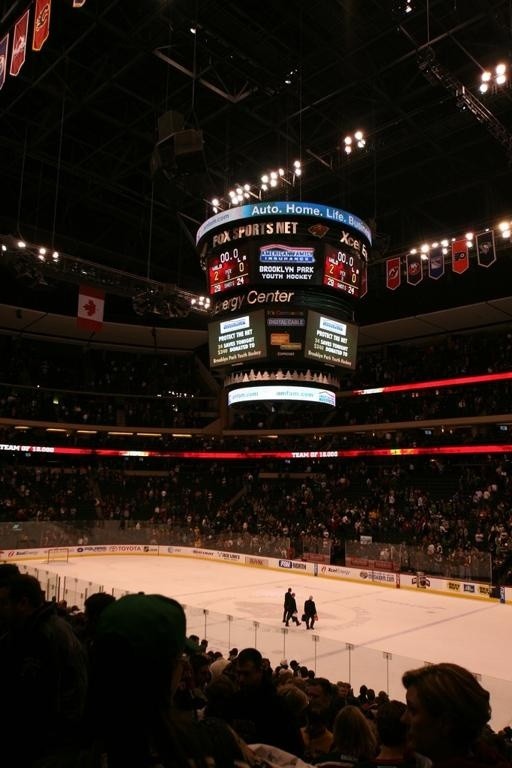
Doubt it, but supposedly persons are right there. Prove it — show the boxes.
[2,335,512,585]
[0,560,511,768]
[303,595,317,629]
[285,592,301,627]
[282,588,292,623]
[0,240,147,300]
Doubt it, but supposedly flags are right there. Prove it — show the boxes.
[75,282,104,335]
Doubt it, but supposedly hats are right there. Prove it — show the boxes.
[101,590,198,653]
